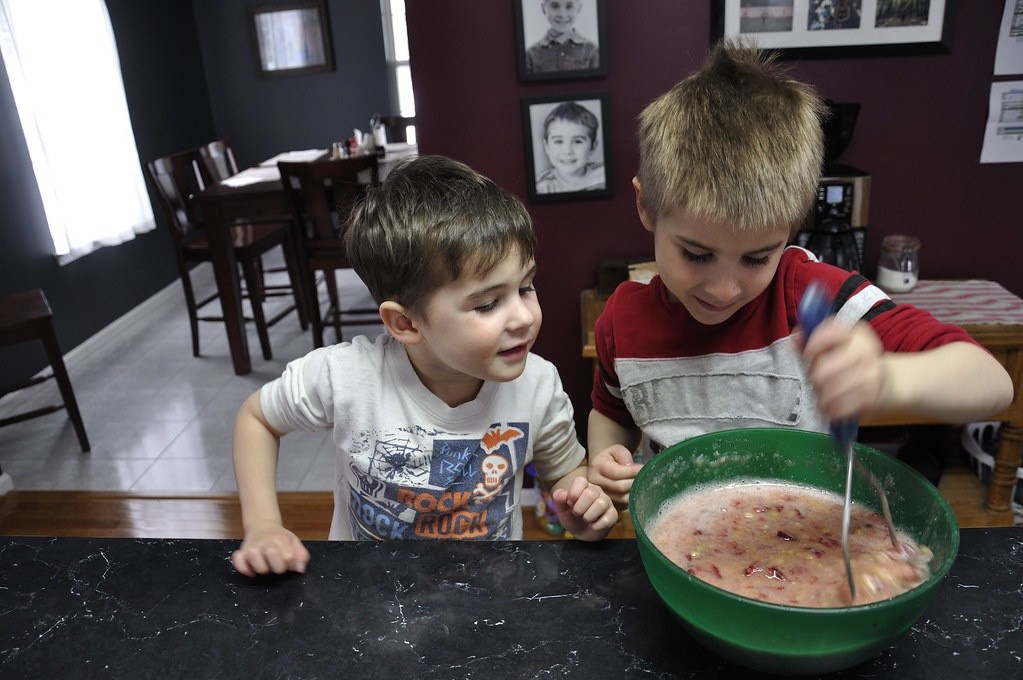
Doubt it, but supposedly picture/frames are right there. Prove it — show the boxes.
[510,0,610,86]
[520,91,615,205]
[709,0,956,64]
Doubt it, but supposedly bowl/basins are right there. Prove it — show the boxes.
[628,427,959,674]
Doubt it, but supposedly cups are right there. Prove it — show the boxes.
[877,236,921,293]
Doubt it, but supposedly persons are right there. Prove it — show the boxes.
[535,102,606,194]
[524,0,599,73]
[232,155,618,578]
[587,44,1014,512]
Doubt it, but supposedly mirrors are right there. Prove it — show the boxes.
[246,0,338,84]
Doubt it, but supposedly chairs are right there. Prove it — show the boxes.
[204,137,296,223]
[380,116,415,143]
[0,286,94,455]
[276,155,383,351]
[144,148,309,361]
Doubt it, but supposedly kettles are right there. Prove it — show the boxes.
[810,214,861,275]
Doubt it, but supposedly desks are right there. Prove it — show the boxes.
[0,530,1023,680]
[580,278,1023,528]
[187,142,418,378]
